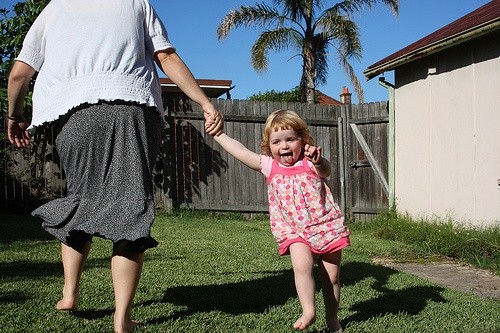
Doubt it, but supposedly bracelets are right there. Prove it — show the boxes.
[8,113,24,120]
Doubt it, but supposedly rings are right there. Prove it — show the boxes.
[15,136,19,138]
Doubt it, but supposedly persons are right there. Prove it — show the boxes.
[7,0,224,333]
[204,109,351,333]
